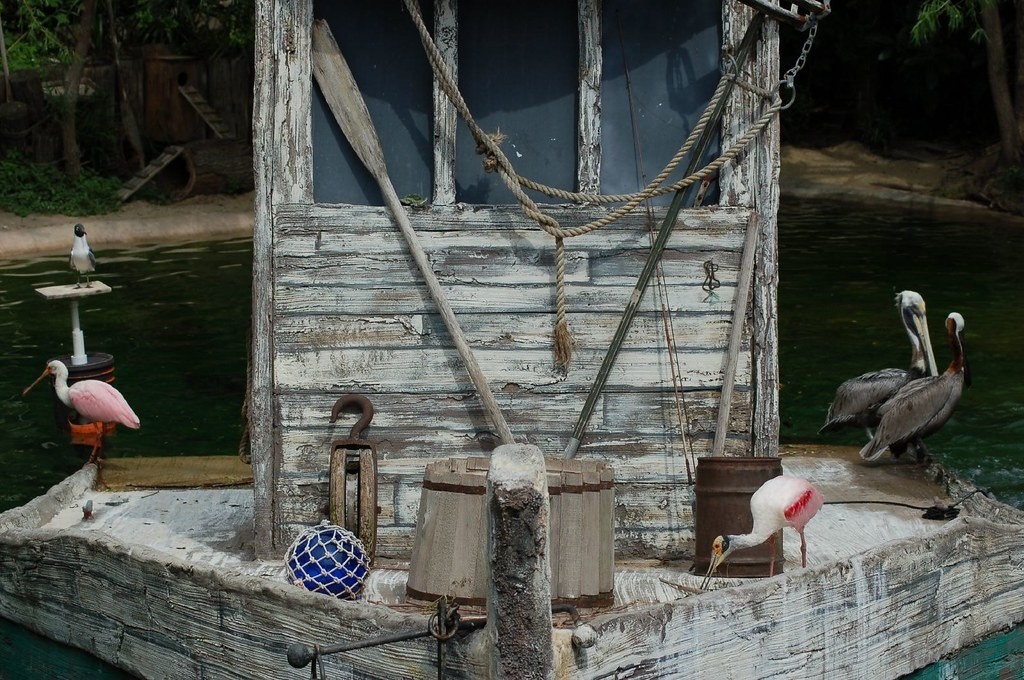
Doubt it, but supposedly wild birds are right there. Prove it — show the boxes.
[24,360,140,489]
[858,311,971,461]
[69,223,97,289]
[700,474,824,589]
[817,288,938,445]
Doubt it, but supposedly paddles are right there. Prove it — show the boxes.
[310,11,521,446]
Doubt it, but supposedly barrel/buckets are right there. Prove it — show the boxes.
[692,457,786,577]
